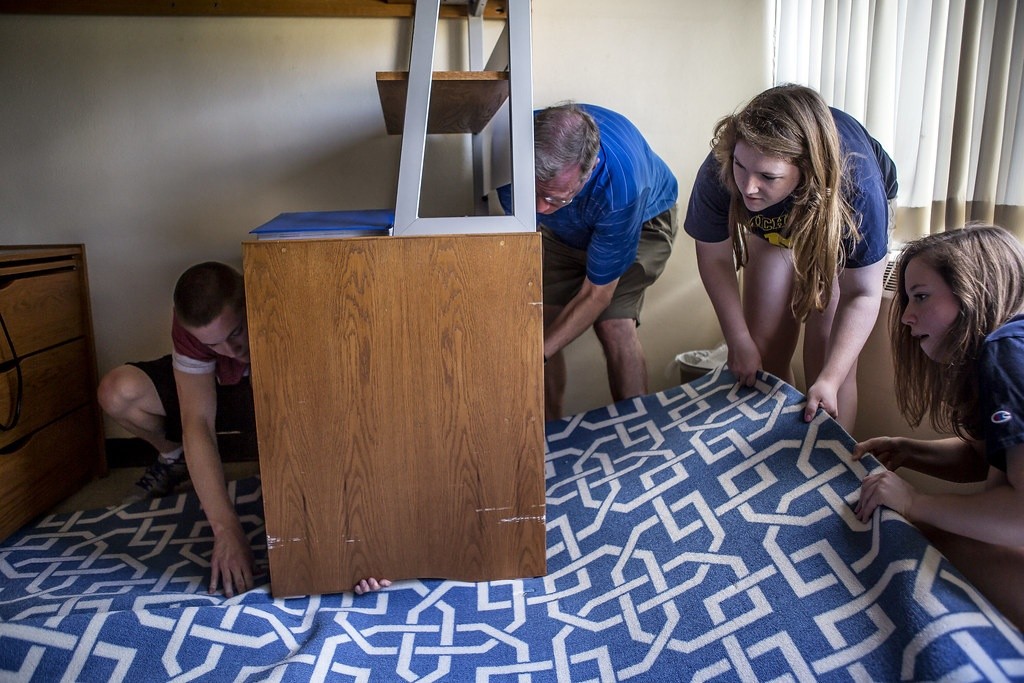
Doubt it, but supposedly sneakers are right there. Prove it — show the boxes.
[130,452,187,499]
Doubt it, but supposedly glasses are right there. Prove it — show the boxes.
[539,195,573,208]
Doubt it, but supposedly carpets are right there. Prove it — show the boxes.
[0,356,1023,682]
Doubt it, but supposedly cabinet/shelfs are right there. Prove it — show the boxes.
[1,241,113,552]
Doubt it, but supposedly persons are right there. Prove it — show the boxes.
[683,83,899,437]
[851,219,1024,632]
[97,262,391,598]
[496,101,678,421]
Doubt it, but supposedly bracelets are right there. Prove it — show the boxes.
[544,355,548,365]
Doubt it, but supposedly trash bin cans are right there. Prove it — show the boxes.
[670,345,734,387]
